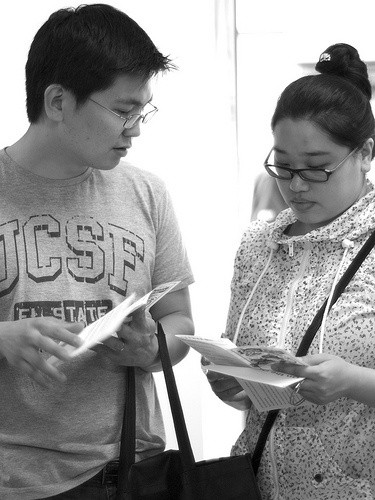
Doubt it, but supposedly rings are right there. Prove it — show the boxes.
[121,342,124,351]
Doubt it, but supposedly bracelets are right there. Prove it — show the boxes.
[150,334,161,367]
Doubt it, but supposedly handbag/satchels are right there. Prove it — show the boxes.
[105,324,195,500]
[196,453,261,500]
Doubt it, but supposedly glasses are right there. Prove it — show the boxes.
[86,93,159,130]
[264,143,362,183]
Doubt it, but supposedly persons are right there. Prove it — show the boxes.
[201,43,375,500]
[0,5,195,500]
